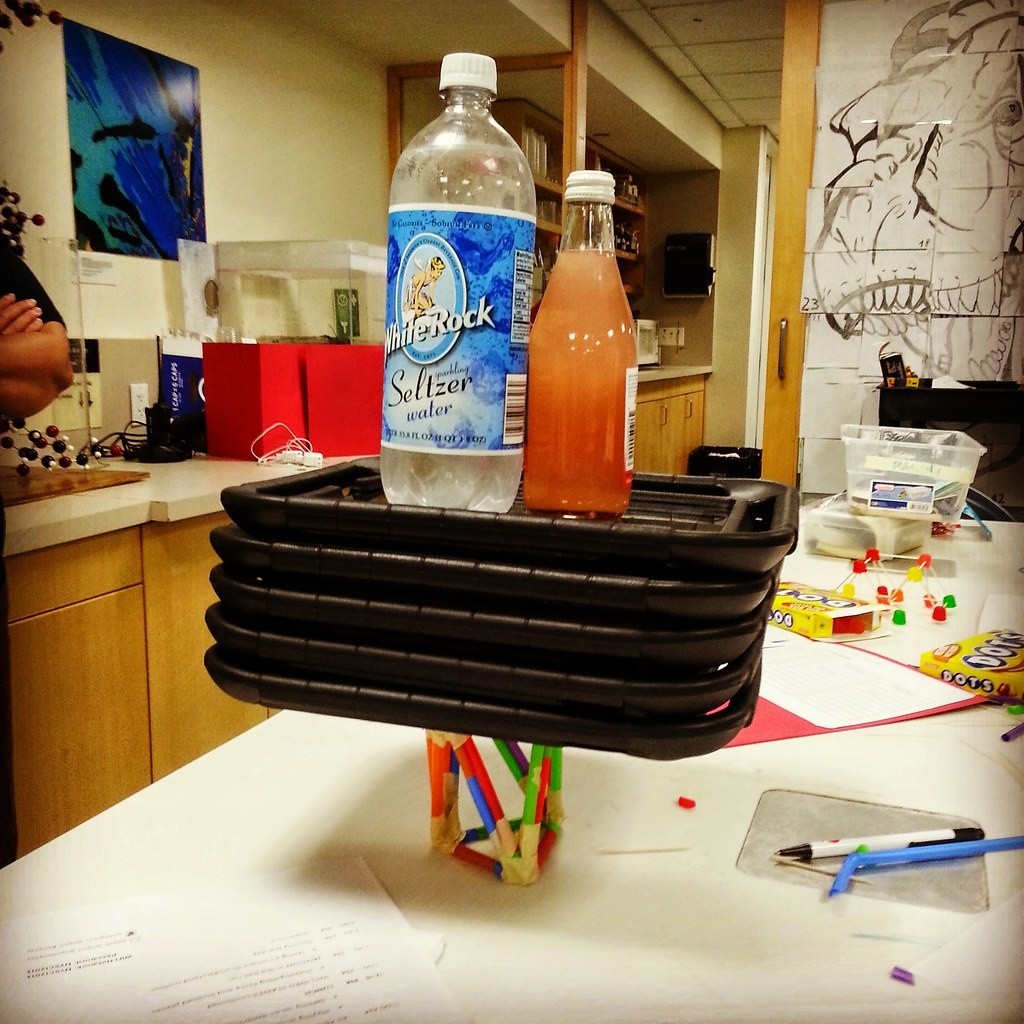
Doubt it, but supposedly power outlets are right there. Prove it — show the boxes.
[129,383,151,427]
[658,327,685,347]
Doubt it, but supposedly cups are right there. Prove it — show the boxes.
[544,201,557,223]
[536,199,543,221]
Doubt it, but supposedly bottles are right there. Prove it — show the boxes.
[530,236,546,332]
[522,169,639,521]
[380,52,536,513]
[547,234,559,285]
[525,126,547,179]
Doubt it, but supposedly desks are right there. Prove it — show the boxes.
[877,374,1024,509]
[0,519,1024,1024]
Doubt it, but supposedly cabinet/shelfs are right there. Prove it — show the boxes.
[488,96,648,306]
[4,507,282,859]
[633,374,706,478]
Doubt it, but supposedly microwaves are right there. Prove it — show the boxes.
[633,319,658,365]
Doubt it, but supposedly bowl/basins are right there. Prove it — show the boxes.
[840,423,988,527]
[800,490,932,561]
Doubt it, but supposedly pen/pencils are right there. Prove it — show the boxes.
[774,827,985,861]
[1002,722,1024,742]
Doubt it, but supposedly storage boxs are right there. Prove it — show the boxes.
[839,422,988,527]
[764,580,892,647]
[632,319,658,366]
[878,342,907,388]
[212,238,388,346]
[296,342,386,458]
[201,341,306,458]
[799,492,933,564]
[920,628,1024,708]
[160,355,204,454]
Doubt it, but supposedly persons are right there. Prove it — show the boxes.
[0,234,75,869]
